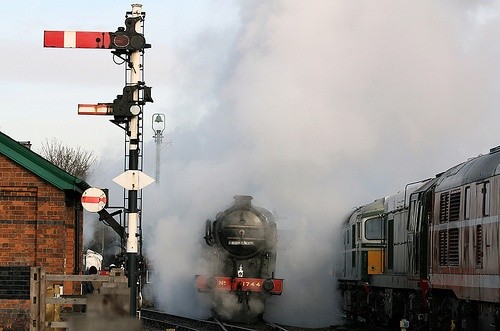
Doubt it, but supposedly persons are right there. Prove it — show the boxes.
[82,266,98,311]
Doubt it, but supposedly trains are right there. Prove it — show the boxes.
[329,144,500,330]
[192,195,285,322]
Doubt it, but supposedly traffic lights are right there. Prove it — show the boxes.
[115,30,146,49]
